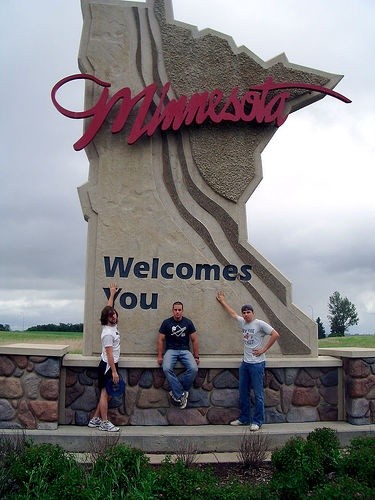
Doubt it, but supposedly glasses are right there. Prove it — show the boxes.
[174,309,182,311]
[242,311,250,314]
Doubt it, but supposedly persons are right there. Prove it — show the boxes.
[216,291,279,431]
[88,282,120,432]
[158,302,199,409]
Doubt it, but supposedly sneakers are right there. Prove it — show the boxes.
[169,390,181,402]
[250,423,259,431]
[98,419,120,431]
[179,391,188,409]
[88,418,102,427]
[230,420,243,426]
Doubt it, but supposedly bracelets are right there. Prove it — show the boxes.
[195,357,199,359]
